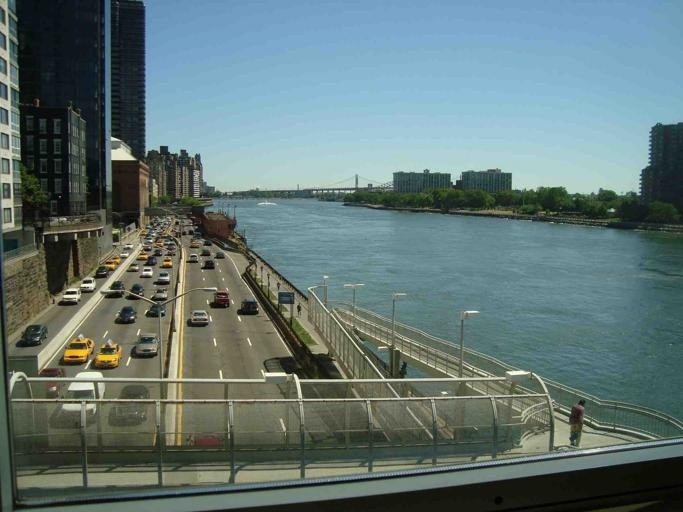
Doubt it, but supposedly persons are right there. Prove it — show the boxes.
[568,398,586,448]
[296,301,302,318]
[276,280,281,290]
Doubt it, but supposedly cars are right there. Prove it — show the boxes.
[194,431,224,446]
[182,224,225,268]
[190,290,259,325]
[21,215,180,426]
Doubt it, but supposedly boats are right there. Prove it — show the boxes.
[257,199,277,206]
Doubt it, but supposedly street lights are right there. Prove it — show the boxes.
[99,287,217,399]
[322,276,365,305]
[391,292,408,348]
[227,203,238,220]
[459,311,480,377]
